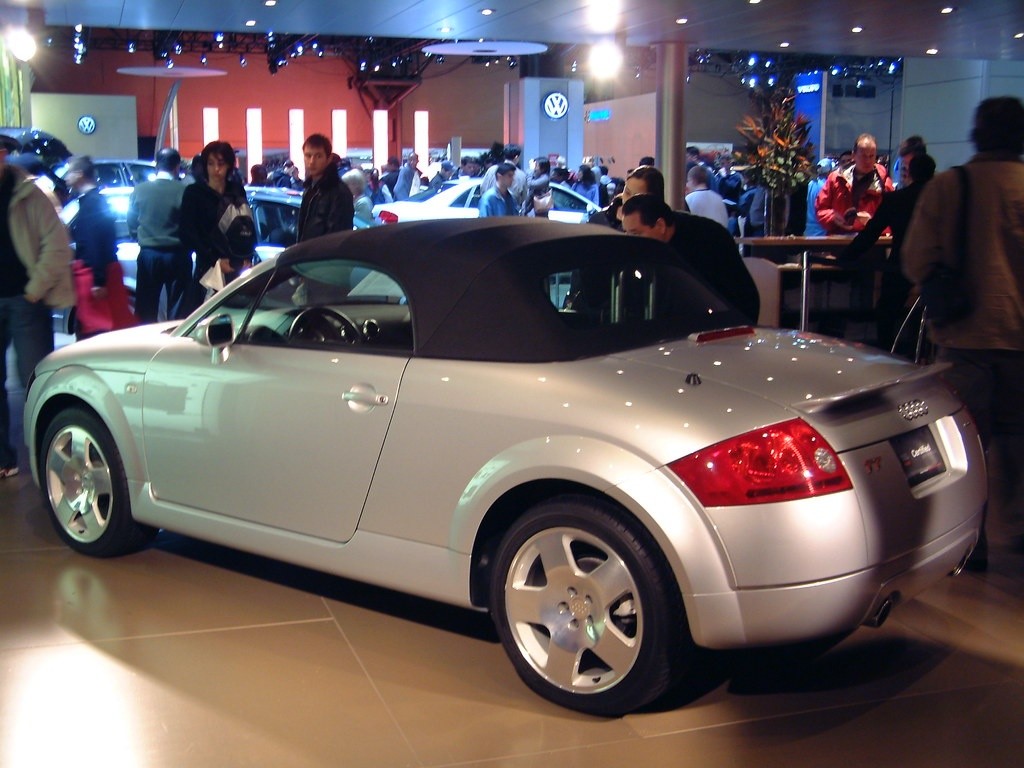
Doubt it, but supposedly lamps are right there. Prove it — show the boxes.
[424,39,517,69]
[686,49,903,89]
[71,24,414,76]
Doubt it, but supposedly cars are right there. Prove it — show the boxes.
[46,158,183,239]
[115,187,379,295]
[371,175,604,229]
[22,216,995,717]
[0,126,74,168]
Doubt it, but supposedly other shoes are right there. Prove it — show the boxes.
[0,465,19,479]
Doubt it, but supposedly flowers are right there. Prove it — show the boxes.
[735,89,821,194]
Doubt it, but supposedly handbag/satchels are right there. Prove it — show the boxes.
[922,165,968,327]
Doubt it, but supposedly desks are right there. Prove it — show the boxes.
[735,235,893,334]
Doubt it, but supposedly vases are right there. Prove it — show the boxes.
[763,188,790,238]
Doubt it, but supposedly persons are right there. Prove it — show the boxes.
[561,164,664,318]
[902,94,1024,571]
[0,133,141,482]
[621,193,765,329]
[838,154,935,365]
[297,132,355,244]
[124,139,260,323]
[250,143,654,239]
[685,131,927,264]
